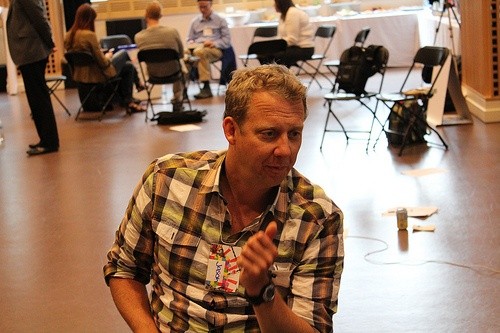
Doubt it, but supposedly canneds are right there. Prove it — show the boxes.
[398,229,408,251]
[396,208,408,230]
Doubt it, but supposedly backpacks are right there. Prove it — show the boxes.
[388,94,427,147]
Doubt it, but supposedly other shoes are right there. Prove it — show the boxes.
[138,85,148,93]
[27,148,53,154]
[128,103,147,115]
[172,88,188,102]
[29,143,42,147]
[194,88,212,98]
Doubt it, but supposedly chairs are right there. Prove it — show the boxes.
[292,26,334,90]
[239,27,277,67]
[138,47,192,122]
[66,51,127,121]
[373,45,449,150]
[29,75,71,120]
[322,29,370,106]
[320,44,389,153]
[196,47,237,97]
[100,35,130,50]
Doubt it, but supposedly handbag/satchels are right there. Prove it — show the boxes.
[152,110,207,124]
[338,49,371,94]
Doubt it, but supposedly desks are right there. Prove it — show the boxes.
[231,9,460,69]
[117,44,135,50]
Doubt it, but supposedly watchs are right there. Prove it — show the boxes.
[244,270,277,306]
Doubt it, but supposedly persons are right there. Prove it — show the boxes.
[180,0,232,99]
[134,1,189,113]
[6,0,60,155]
[61,3,147,113]
[273,0,317,68]
[102,63,344,333]
